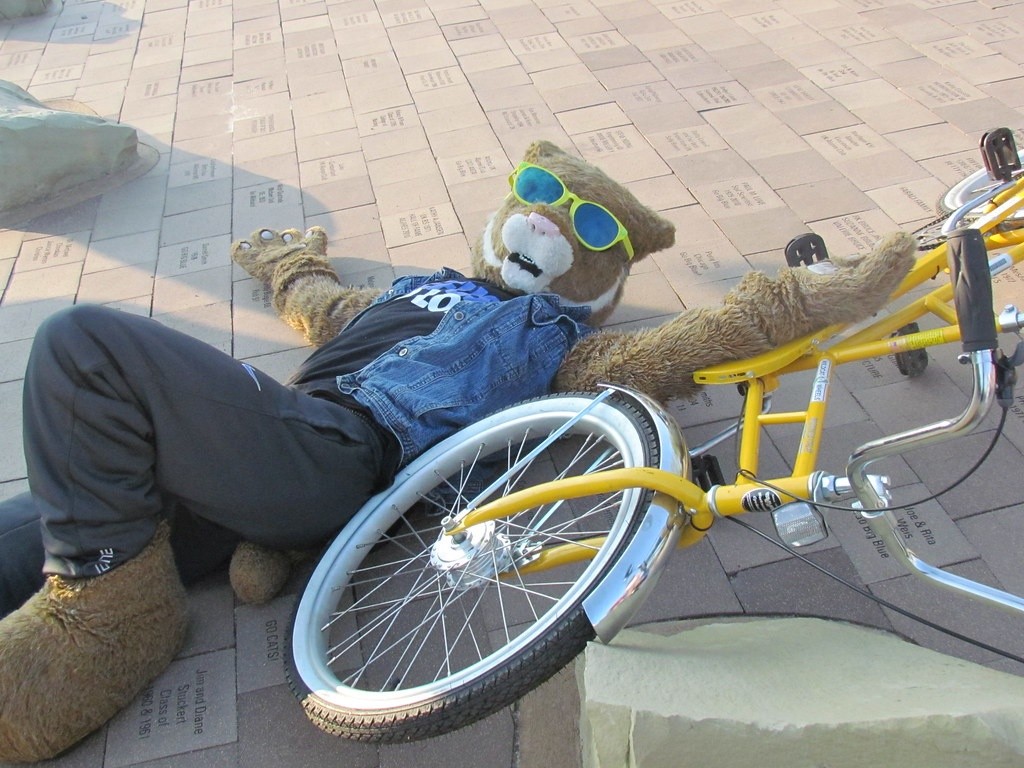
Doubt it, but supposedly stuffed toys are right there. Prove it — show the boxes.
[0,138,914,764]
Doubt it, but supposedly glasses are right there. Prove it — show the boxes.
[511,162,638,263]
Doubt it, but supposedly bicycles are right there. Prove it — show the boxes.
[283,126,1023,745]
